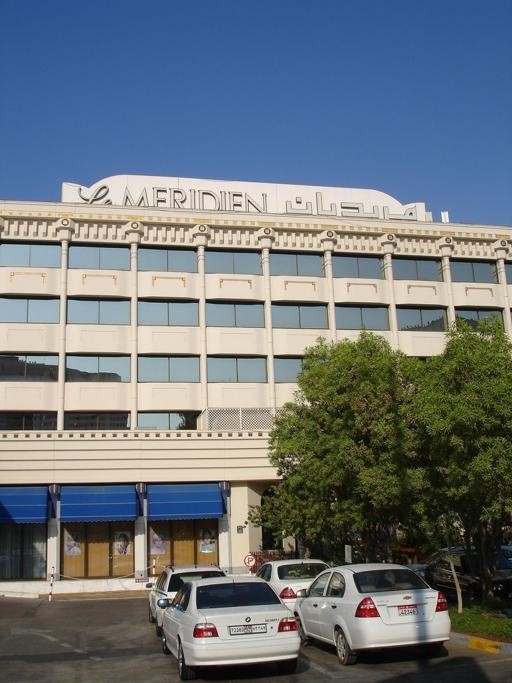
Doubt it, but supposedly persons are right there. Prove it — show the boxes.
[150,532,165,553]
[65,534,80,555]
[115,533,128,555]
[200,529,215,551]
